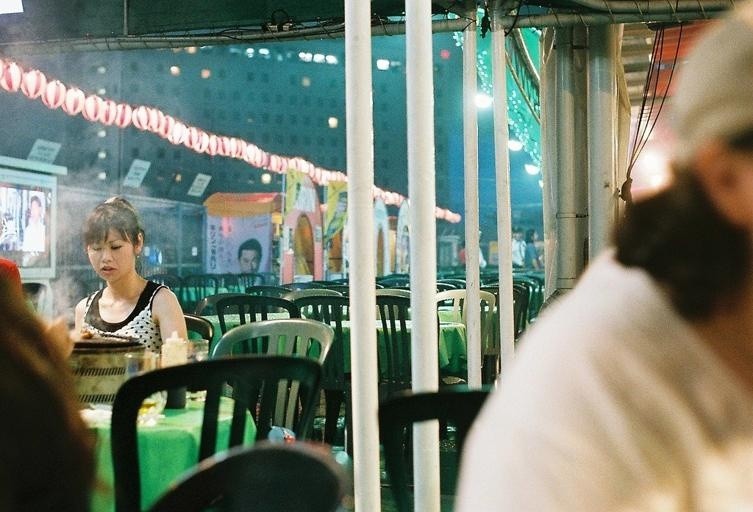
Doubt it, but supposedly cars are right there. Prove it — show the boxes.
[124,351,160,416]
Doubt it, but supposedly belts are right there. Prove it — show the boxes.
[186,338,210,402]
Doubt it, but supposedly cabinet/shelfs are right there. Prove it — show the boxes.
[326,192,349,274]
[161,330,188,409]
[399,226,411,274]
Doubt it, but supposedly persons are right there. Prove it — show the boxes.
[523,227,542,270]
[511,224,527,269]
[459,238,466,265]
[23,193,46,251]
[75,195,189,362]
[445,0,751,511]
[237,237,264,274]
[0,270,102,511]
[478,230,488,268]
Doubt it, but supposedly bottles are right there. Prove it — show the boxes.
[670,8,753,173]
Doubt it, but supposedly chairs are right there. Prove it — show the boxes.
[73,270,545,512]
